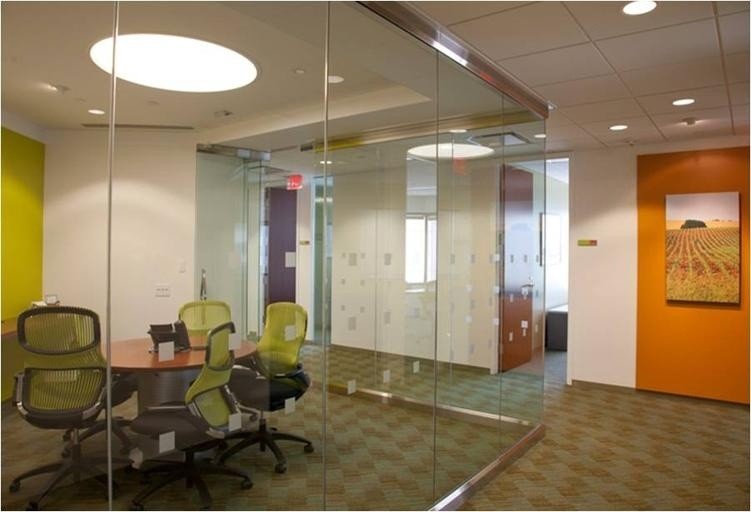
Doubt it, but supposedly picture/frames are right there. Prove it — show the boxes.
[96,336,257,417]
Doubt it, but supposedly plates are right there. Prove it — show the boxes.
[546,305,568,352]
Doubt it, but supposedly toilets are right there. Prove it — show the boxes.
[147,320,190,353]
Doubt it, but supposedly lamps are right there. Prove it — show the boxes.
[60,374,135,458]
[218,303,314,473]
[9,307,117,511]
[179,300,258,422]
[124,321,253,511]
